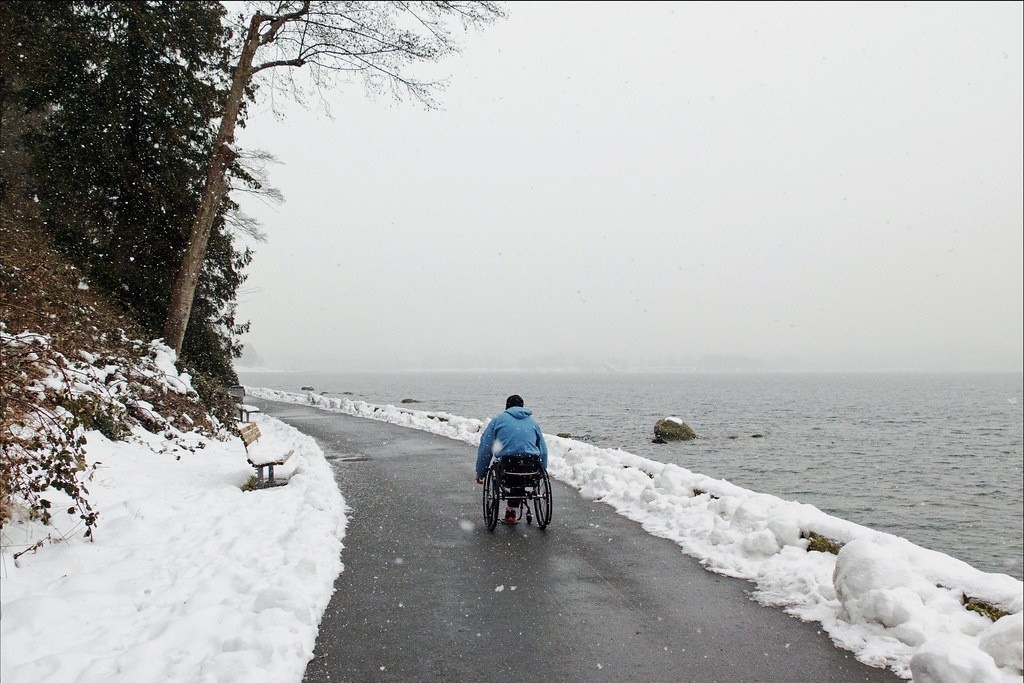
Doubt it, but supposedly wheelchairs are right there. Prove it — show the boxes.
[476,452,553,533]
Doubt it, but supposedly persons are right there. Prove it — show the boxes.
[475,394,547,523]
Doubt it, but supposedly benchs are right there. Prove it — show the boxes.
[229,387,264,422]
[238,422,299,485]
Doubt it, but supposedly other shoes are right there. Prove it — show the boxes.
[505,507,517,522]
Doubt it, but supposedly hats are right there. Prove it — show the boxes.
[506,395,523,410]
